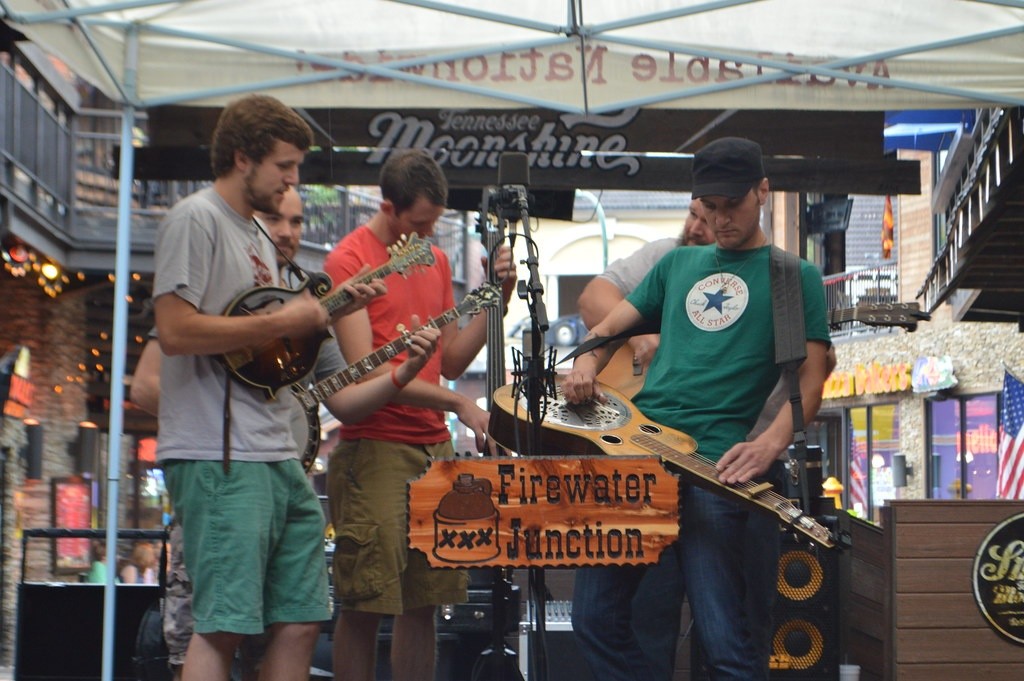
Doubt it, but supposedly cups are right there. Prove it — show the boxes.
[839,665,861,681]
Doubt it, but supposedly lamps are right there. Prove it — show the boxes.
[18,423,43,483]
[67,422,98,479]
[892,453,913,487]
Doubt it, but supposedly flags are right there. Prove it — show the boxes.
[880,195,896,260]
[995,368,1024,502]
[847,413,867,509]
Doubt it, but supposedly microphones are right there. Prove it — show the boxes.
[497,152,528,248]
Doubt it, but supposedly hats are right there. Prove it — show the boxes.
[691,137,766,200]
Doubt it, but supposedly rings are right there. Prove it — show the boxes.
[597,392,607,401]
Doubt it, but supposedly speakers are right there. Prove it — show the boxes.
[692,531,840,681]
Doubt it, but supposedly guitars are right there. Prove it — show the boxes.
[288,280,500,471]
[594,300,933,402]
[477,184,509,456]
[219,231,437,398]
[489,368,838,553]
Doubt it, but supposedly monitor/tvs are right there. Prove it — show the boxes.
[805,198,854,234]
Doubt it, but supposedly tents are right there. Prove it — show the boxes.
[0,0,1023,681]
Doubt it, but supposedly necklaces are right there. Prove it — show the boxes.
[715,234,768,289]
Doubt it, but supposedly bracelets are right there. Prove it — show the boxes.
[391,368,406,388]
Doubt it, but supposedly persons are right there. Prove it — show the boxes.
[118,539,154,587]
[84,543,123,585]
[559,134,832,681]
[128,174,444,681]
[575,191,838,681]
[319,149,516,681]
[149,542,172,586]
[151,95,332,681]
